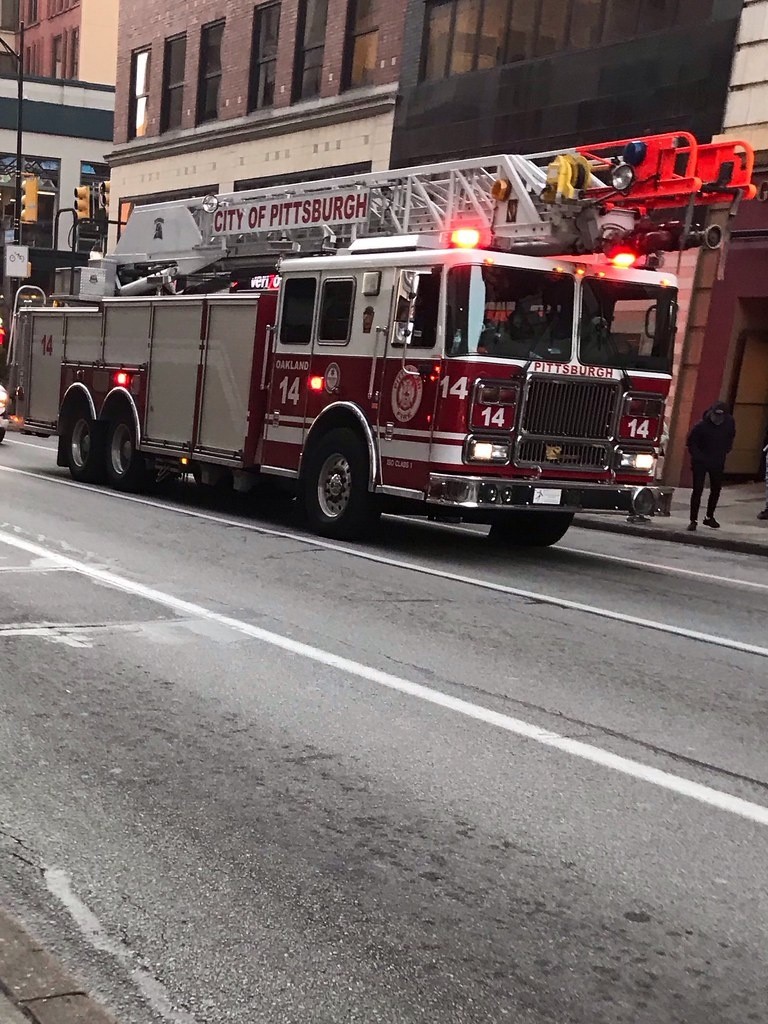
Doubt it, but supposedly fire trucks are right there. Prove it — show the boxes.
[17,126,758,546]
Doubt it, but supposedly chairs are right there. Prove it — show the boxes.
[508,295,549,342]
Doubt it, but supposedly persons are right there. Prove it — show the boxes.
[757,439,768,520]
[686,402,736,531]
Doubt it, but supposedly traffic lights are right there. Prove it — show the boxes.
[73,184,91,221]
[99,180,110,218]
[19,173,38,223]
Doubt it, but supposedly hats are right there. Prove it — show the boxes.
[710,401,727,424]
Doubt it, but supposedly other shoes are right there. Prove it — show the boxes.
[702,516,720,528]
[686,521,698,531]
[757,509,768,519]
